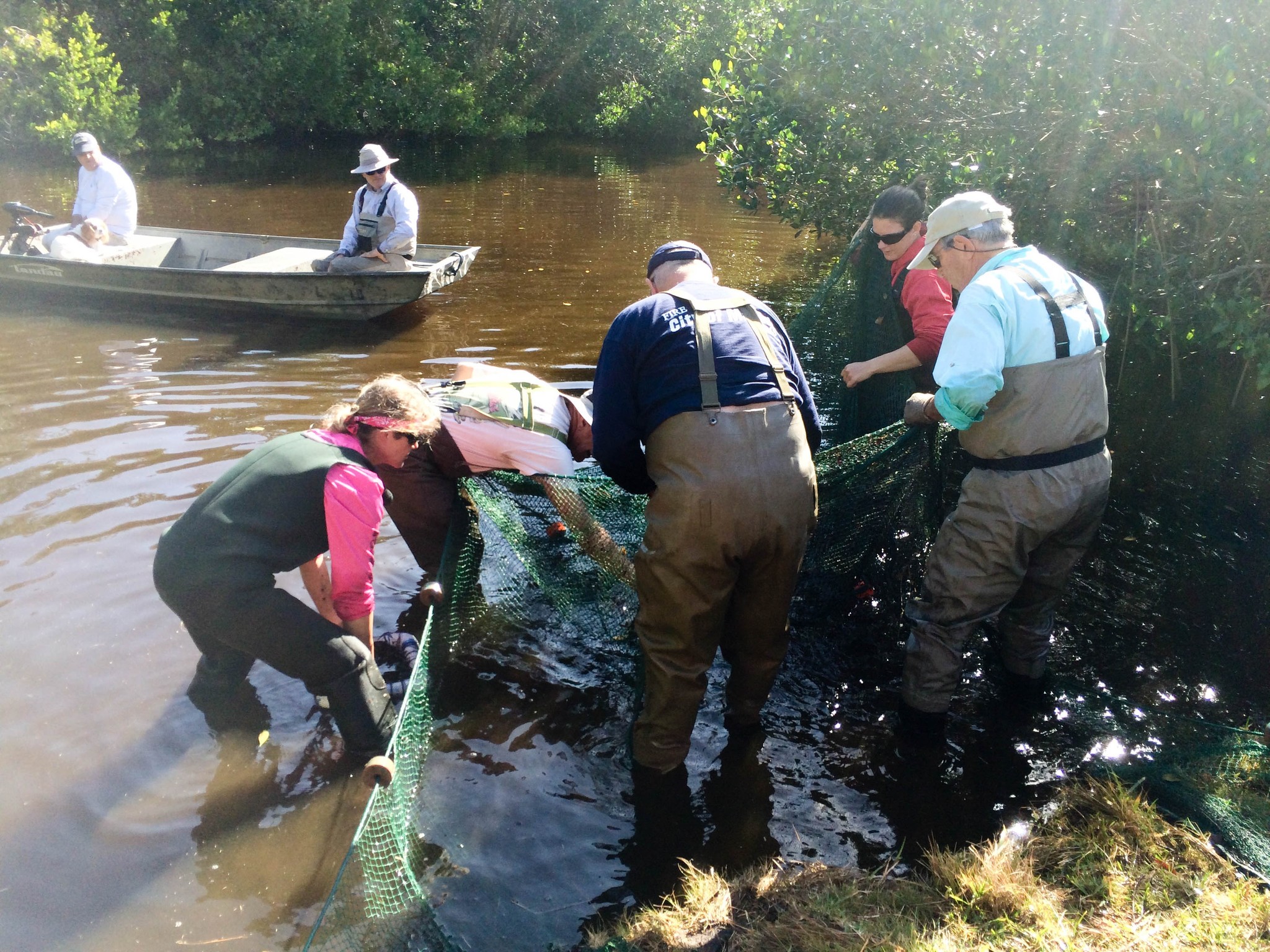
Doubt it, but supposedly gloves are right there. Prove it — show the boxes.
[903,392,935,424]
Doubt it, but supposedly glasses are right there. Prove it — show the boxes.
[927,234,973,268]
[365,167,386,175]
[377,429,418,446]
[870,225,913,245]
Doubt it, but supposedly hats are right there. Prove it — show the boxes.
[350,144,399,173]
[906,191,1007,270]
[647,240,713,280]
[559,388,594,426]
[72,132,97,155]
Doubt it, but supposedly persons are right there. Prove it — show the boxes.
[42,132,137,260]
[311,143,419,273]
[841,182,959,388]
[373,363,638,581]
[894,192,1114,732]
[154,373,441,757]
[593,240,824,774]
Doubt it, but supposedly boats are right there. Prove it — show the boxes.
[0,201,482,320]
